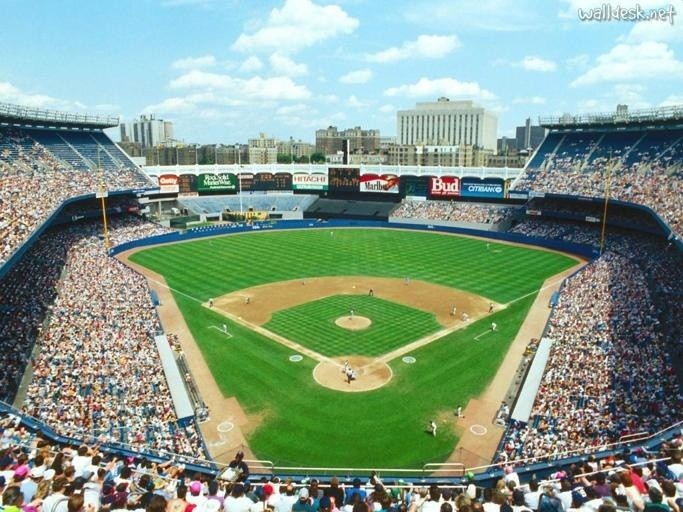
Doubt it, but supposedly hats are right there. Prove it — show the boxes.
[298,488,308,501]
[263,486,272,494]
[591,473,604,480]
[14,467,27,476]
[190,483,200,494]
[30,467,44,479]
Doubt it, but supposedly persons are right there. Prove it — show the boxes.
[0,125,683,512]
